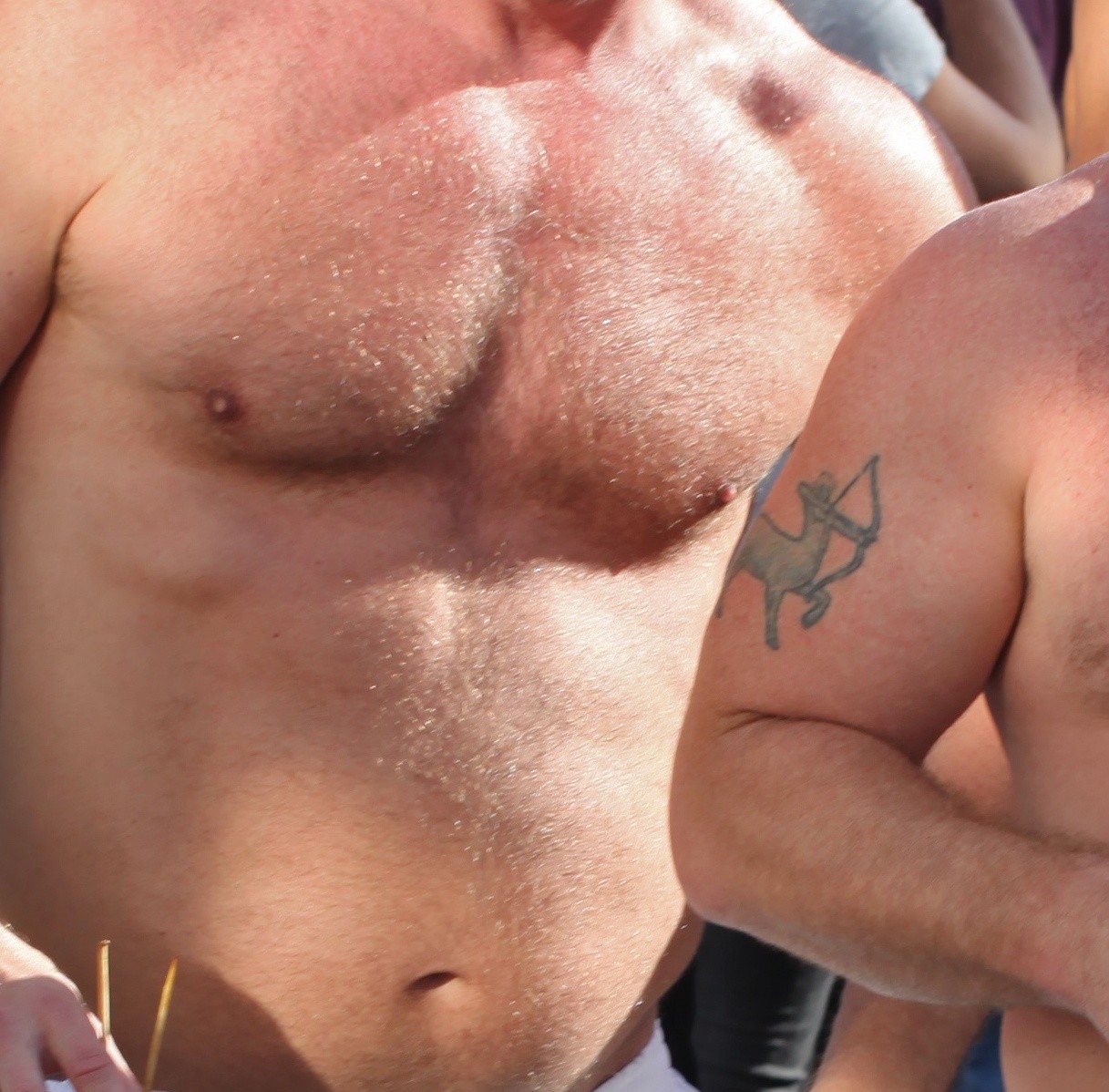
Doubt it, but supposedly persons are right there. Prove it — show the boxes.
[1,0,1023,1092]
[777,0,1069,205]
[669,153,1109,1092]
